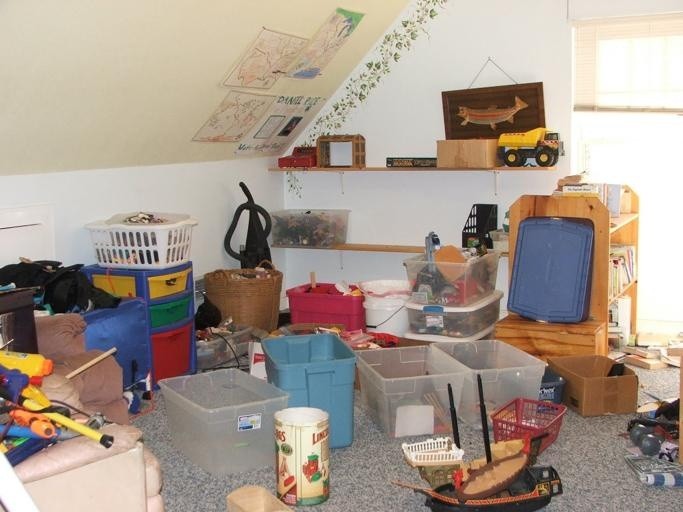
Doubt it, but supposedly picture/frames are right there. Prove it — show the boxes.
[441,81,545,140]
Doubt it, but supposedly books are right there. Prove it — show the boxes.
[561,184,680,370]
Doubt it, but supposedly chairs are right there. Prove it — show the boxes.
[0,377,163,512]
[31,313,128,424]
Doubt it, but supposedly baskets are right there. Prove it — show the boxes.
[84,211,199,268]
[206,260,283,332]
[490,397,567,455]
[462,204,497,248]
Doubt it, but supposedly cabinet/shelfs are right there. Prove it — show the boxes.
[81,262,197,388]
[496,182,639,363]
[266,166,559,262]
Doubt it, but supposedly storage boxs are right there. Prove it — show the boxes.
[545,353,641,417]
[401,251,500,304]
[354,345,468,441]
[430,338,549,425]
[434,138,500,170]
[157,367,292,482]
[266,209,352,247]
[259,333,358,452]
[285,282,365,333]
[540,373,567,413]
[405,293,505,337]
[197,323,253,369]
